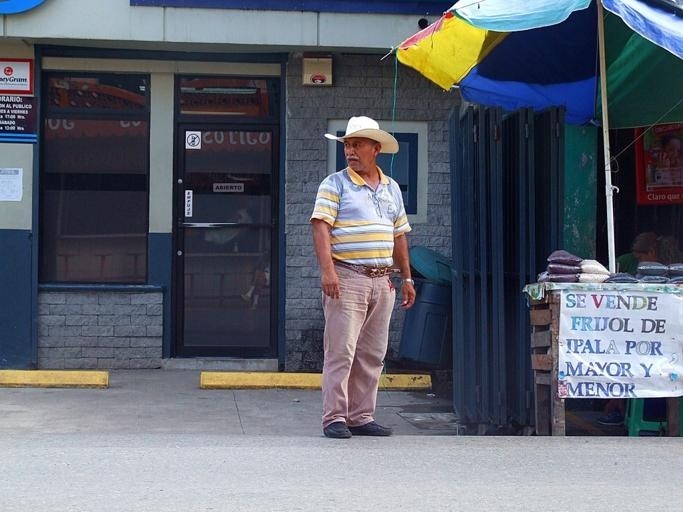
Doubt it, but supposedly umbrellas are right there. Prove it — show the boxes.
[380,0,682,276]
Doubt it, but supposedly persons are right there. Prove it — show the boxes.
[595,251,642,426]
[647,131,682,186]
[631,231,683,269]
[236,252,273,309]
[308,115,417,438]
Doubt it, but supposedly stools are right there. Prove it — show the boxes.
[622,399,683,436]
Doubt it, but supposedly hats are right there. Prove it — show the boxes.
[324,116,399,154]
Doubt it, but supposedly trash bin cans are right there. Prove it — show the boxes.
[399,245,453,370]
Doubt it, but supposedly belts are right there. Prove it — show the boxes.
[335,260,401,278]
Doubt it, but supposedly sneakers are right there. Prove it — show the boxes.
[241,294,256,311]
[597,409,624,426]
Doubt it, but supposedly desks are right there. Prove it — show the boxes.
[522,283,683,436]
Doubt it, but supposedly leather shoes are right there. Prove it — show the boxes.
[348,421,392,436]
[324,422,352,438]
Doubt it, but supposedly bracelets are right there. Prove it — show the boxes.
[401,278,414,285]
